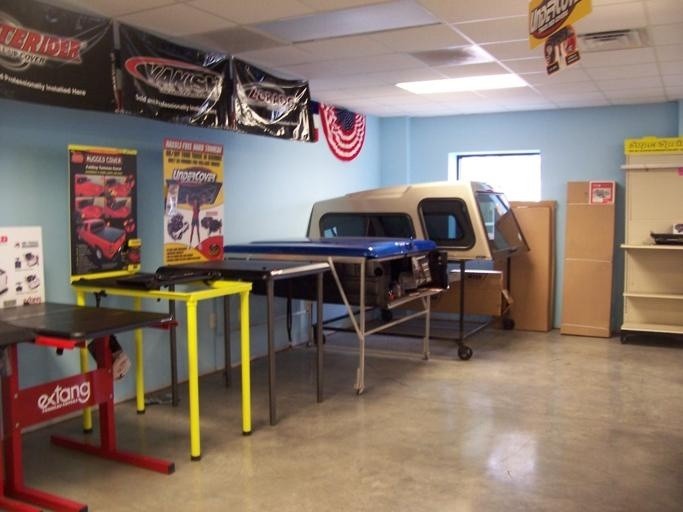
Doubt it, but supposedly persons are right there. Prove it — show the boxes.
[187,196,204,250]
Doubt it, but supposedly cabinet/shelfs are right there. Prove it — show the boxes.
[621,152,683,342]
